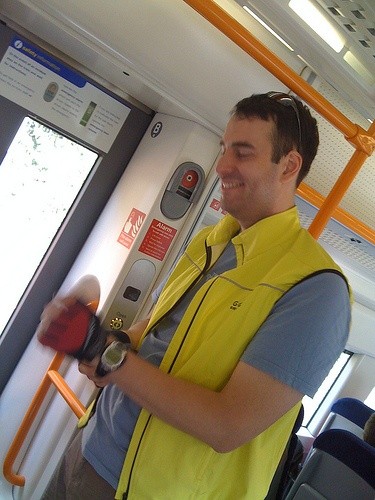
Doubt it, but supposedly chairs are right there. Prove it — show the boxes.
[285,397,374,500]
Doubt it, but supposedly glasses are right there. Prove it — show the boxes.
[266,91,304,155]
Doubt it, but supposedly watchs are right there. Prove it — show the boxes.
[92,334,134,379]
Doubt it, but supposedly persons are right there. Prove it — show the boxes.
[37,92,356,499]
